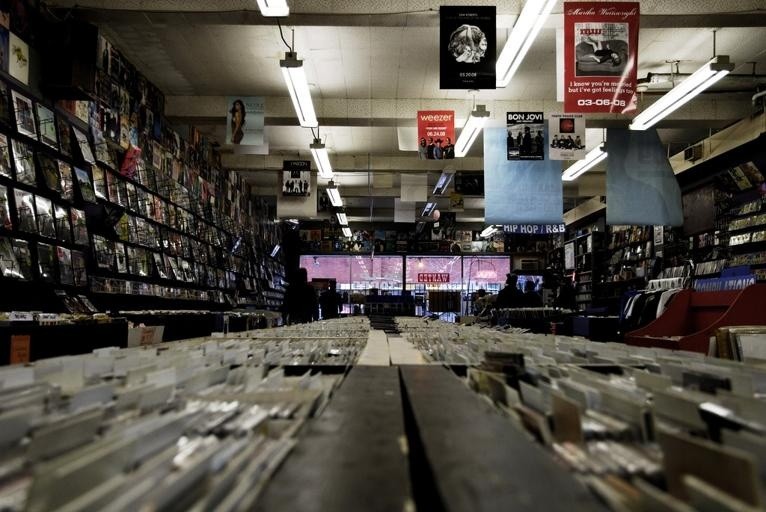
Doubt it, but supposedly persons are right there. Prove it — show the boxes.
[471,273,576,338]
[550,135,585,149]
[581,36,622,67]
[319,280,343,320]
[285,180,309,193]
[229,99,245,144]
[418,138,454,159]
[508,126,544,156]
[281,266,319,326]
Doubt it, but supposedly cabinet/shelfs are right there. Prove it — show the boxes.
[0,11,291,366]
[548,133,765,362]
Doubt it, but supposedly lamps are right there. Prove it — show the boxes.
[255,0,355,243]
[560,126,608,183]
[419,200,438,219]
[480,222,502,240]
[495,1,556,89]
[432,163,457,197]
[628,29,736,134]
[451,90,491,158]
